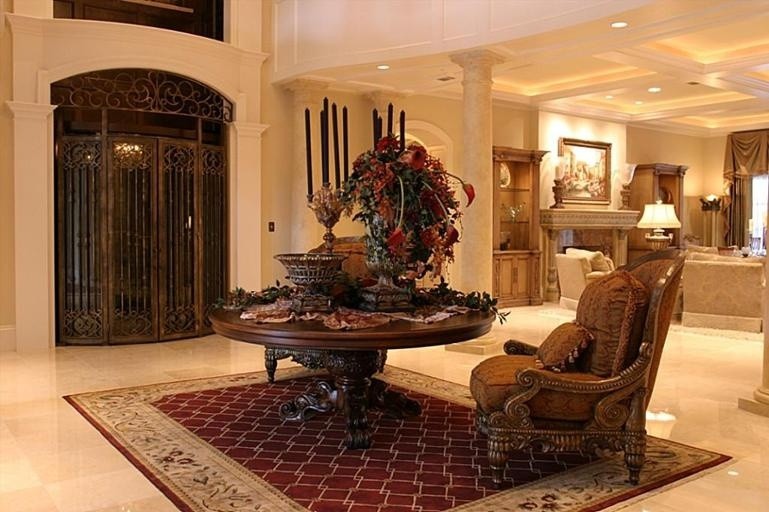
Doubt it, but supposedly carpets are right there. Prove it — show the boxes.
[61,364,737,512]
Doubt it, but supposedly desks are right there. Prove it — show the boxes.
[207,306,495,450]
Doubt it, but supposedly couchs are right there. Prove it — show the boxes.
[555,253,612,312]
[682,260,764,333]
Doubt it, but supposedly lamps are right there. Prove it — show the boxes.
[618,163,637,210]
[550,155,568,209]
[699,194,721,247]
[645,411,676,439]
[637,200,683,251]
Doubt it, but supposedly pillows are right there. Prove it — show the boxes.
[690,252,759,263]
[604,257,615,272]
[687,245,719,260]
[566,248,609,270]
[534,322,594,372]
[578,270,649,377]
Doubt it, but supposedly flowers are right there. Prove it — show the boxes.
[500,198,526,219]
[201,277,512,328]
[341,134,475,283]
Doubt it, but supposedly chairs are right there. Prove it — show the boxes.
[266,237,387,383]
[470,251,685,490]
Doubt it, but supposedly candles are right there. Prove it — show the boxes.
[377,115,382,139]
[373,108,378,149]
[388,102,393,136]
[342,106,347,182]
[748,218,753,234]
[323,97,329,183]
[332,102,341,191]
[400,110,405,151]
[304,108,312,194]
[320,110,323,151]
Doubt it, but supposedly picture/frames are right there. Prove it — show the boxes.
[558,137,611,205]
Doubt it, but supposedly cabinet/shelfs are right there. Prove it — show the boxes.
[627,163,690,265]
[492,146,549,308]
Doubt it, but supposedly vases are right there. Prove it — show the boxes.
[358,261,416,314]
[510,215,516,223]
